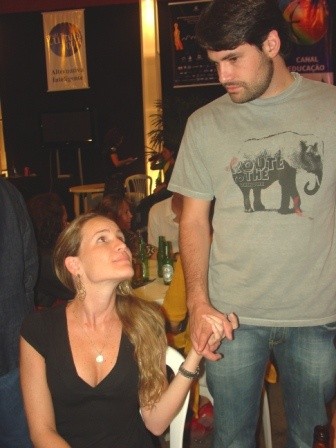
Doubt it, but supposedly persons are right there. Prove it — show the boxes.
[18,212,239,448]
[0,176,39,447]
[167,0,336,448]
[31,139,277,419]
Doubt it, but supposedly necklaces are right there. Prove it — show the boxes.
[85,321,113,363]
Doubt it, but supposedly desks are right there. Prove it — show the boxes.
[70,184,106,217]
[8,174,36,196]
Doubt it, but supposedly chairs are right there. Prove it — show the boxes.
[124,174,153,204]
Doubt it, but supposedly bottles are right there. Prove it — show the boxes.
[10,160,16,177]
[157,236,166,278]
[131,239,144,287]
[163,241,174,285]
[312,425,329,448]
[136,231,149,282]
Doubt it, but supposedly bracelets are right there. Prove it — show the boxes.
[178,362,200,380]
